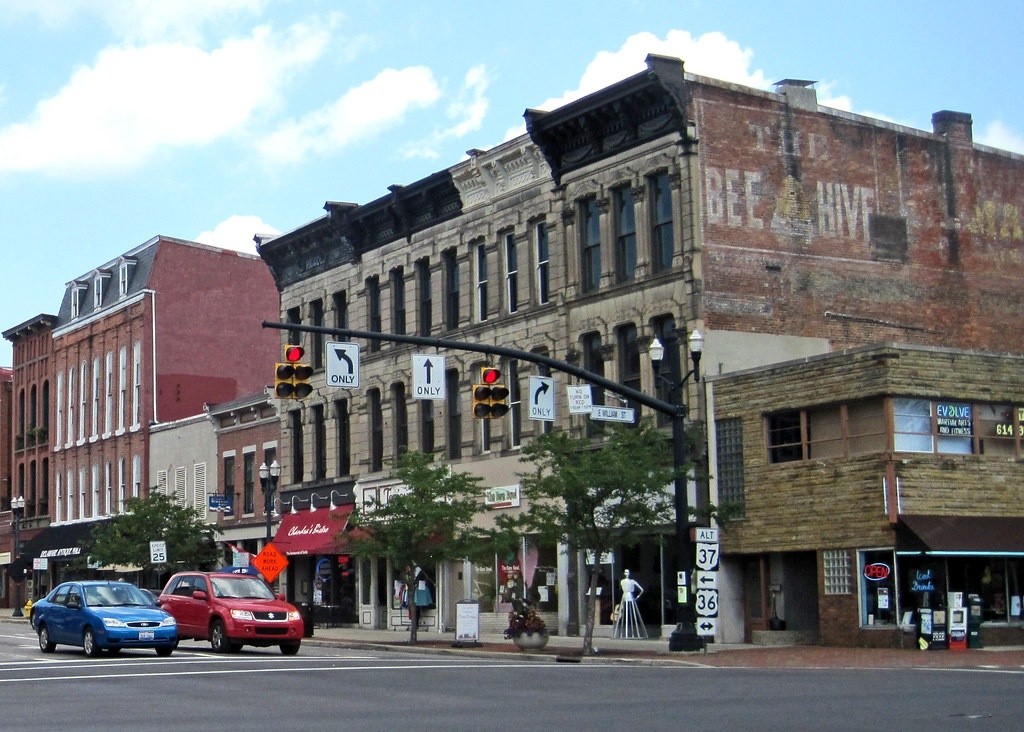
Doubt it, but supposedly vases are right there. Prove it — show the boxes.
[514,630,549,654]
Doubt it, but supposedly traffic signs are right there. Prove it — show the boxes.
[527,375,555,421]
[411,354,446,400]
[589,405,635,424]
[325,341,360,390]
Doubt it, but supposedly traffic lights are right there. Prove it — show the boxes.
[274,345,313,399]
[472,367,509,418]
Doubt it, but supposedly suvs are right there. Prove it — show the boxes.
[156,571,303,654]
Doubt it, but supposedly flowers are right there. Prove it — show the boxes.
[504,605,552,641]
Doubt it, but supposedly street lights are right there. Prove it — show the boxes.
[10,496,25,617]
[649,329,714,652]
[258,460,281,593]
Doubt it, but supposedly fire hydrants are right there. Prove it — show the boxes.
[24,599,33,618]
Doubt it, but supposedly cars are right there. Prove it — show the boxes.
[30,580,178,659]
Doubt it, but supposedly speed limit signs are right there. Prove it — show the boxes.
[150,541,167,563]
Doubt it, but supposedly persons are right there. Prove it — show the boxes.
[620,569,644,601]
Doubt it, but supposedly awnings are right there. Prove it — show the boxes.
[273,504,356,555]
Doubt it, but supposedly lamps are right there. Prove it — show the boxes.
[291,495,309,514]
[273,498,290,517]
[310,493,328,512]
[329,490,348,511]
